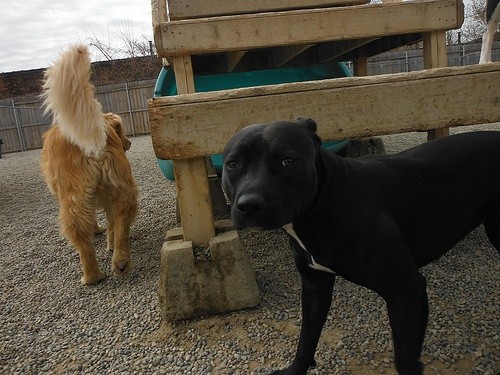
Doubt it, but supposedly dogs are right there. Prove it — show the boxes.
[221,117,500,375]
[37,43,139,286]
